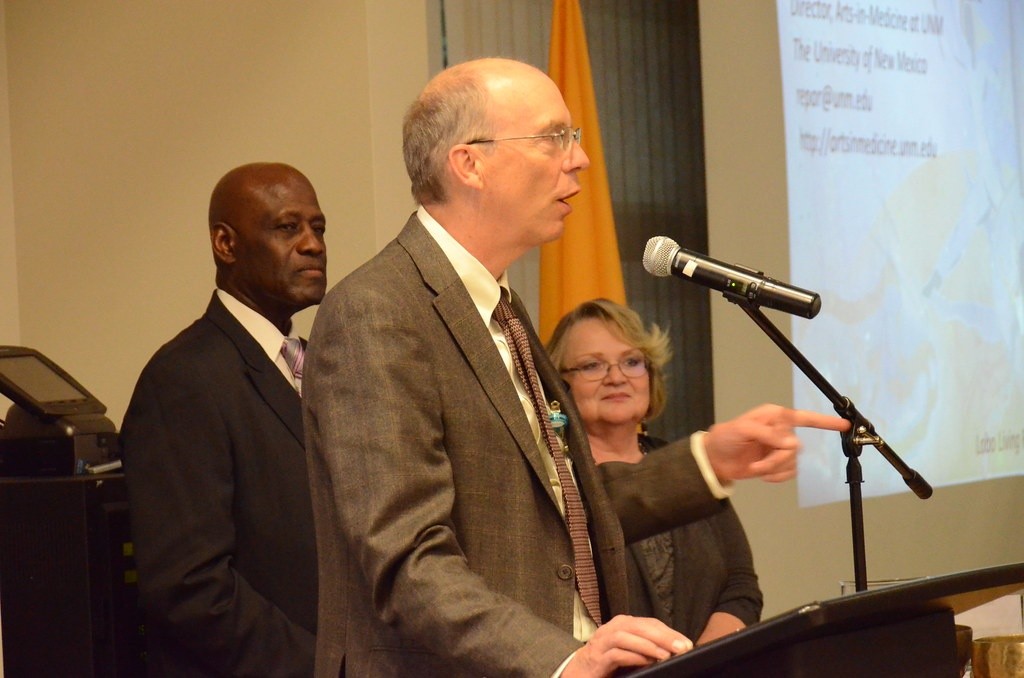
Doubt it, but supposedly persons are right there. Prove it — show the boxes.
[548,298,763,653]
[120,162,345,676]
[303,57,850,678]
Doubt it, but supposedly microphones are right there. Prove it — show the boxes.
[642,234,822,319]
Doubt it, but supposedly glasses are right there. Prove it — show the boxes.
[459,123,587,158]
[550,343,652,391]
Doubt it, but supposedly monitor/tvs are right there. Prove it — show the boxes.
[0,344,108,422]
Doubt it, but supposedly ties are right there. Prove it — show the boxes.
[497,296,605,627]
[281,335,311,389]
